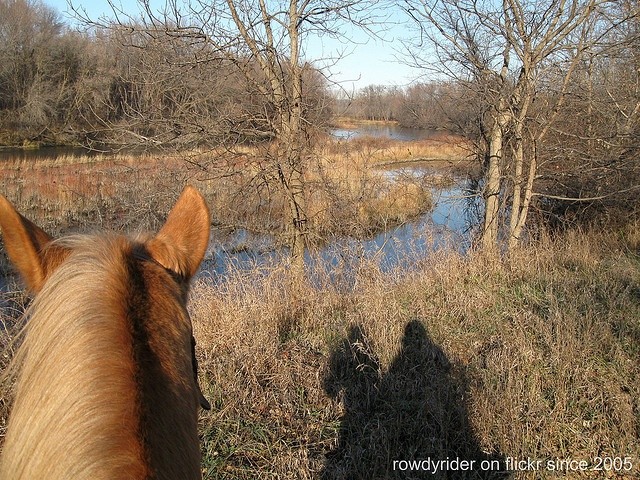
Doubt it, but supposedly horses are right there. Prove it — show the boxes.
[0,181,213,479]
